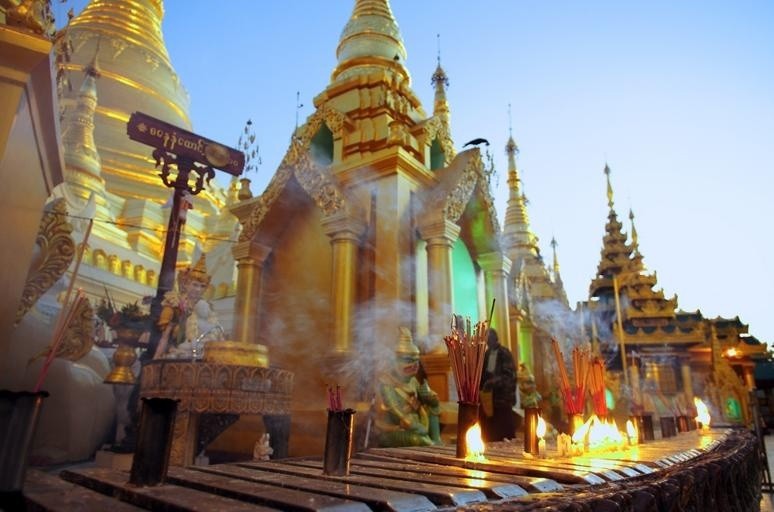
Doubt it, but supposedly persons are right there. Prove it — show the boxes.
[157,252,219,360]
[479,328,518,442]
[372,334,440,446]
